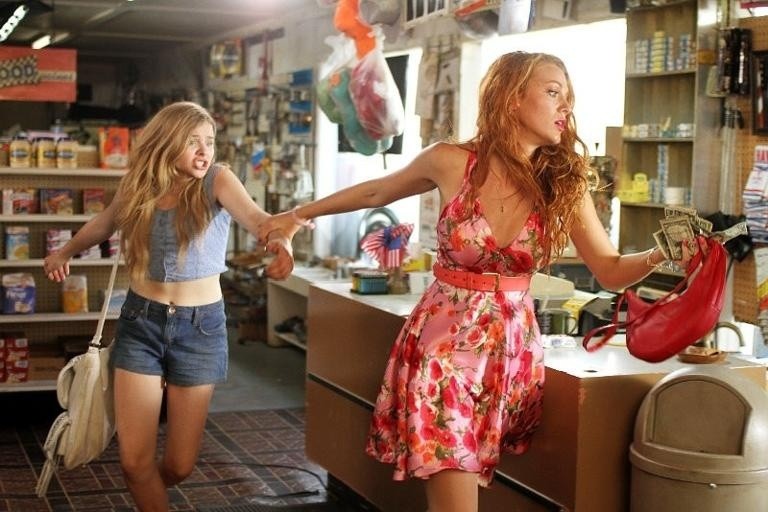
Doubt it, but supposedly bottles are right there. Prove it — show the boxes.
[349,269,410,295]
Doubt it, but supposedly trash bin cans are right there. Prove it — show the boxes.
[629,363,768,512]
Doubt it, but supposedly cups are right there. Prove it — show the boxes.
[540,308,577,336]
[664,186,686,206]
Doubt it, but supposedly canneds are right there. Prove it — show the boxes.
[8,136,77,170]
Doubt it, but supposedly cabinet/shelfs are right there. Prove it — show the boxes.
[617,1,724,279]
[0,144,169,424]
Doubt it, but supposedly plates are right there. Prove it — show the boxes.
[675,349,726,364]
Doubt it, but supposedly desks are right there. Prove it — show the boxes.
[265,259,348,351]
[306,275,765,512]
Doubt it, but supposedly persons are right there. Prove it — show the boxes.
[256,50,709,512]
[40,100,296,512]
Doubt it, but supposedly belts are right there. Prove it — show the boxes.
[434,261,529,292]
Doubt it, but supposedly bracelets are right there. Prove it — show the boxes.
[647,246,664,269]
[290,205,313,228]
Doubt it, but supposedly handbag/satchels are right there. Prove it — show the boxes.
[37,329,117,499]
[625,238,728,362]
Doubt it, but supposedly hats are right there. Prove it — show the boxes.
[333,1,375,59]
[360,1,403,43]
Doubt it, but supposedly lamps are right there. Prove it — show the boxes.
[30,1,133,51]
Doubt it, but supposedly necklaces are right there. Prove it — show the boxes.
[490,166,522,218]
